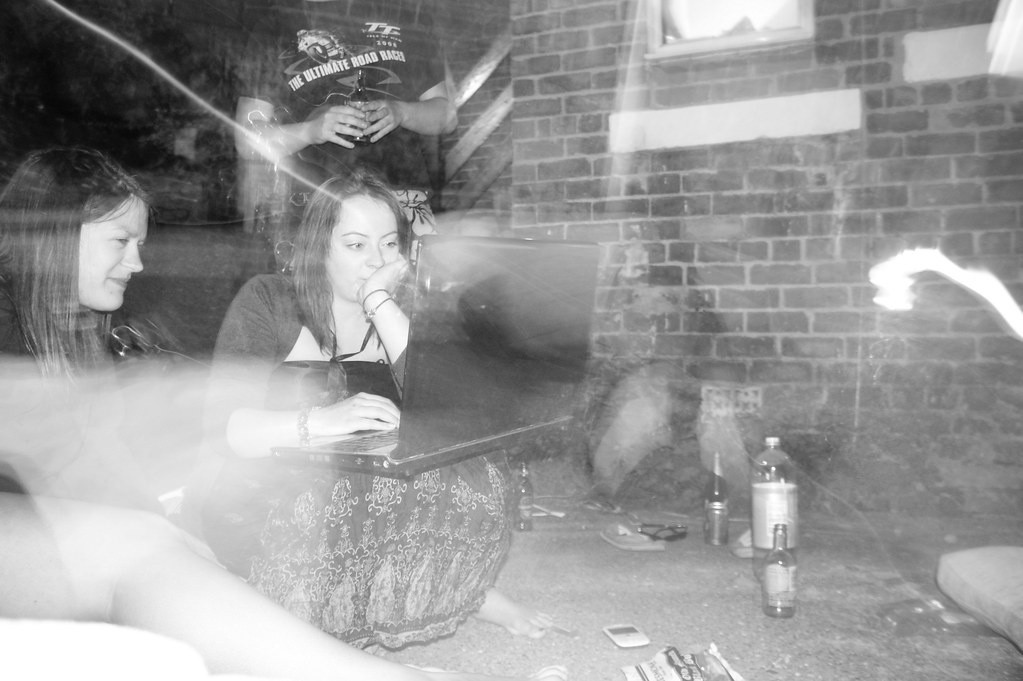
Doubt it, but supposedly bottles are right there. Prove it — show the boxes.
[755,523,799,618]
[513,462,533,532]
[749,435,798,581]
[343,70,373,145]
[702,451,731,547]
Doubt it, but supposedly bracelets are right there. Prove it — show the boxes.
[298,405,312,441]
[367,298,397,323]
[361,288,391,313]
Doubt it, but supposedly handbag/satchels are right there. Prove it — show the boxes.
[111,314,214,369]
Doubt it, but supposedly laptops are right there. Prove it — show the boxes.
[266,236,602,481]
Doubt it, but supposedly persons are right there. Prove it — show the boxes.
[186,167,555,638]
[1,144,569,681]
[235,1,457,262]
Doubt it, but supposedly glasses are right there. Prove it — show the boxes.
[639,523,687,542]
